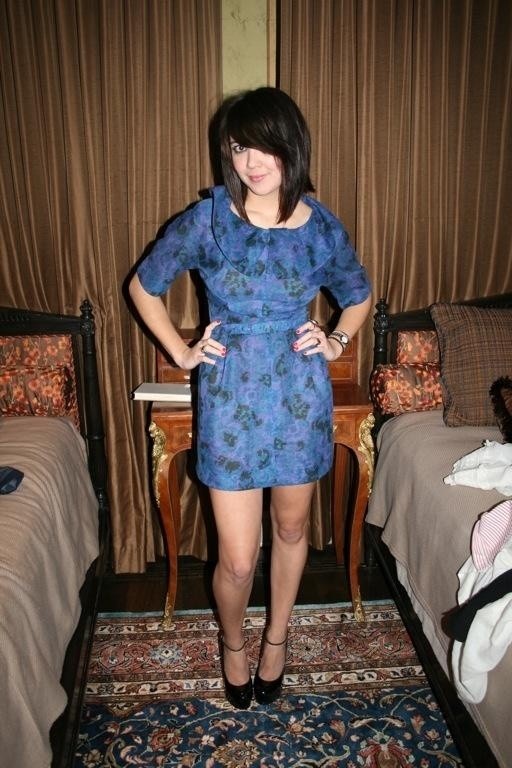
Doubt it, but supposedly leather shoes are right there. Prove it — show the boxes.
[252,627,288,706]
[218,631,252,710]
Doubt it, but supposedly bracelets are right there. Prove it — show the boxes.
[326,335,346,354]
[333,329,352,344]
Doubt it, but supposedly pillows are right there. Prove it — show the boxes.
[0,361,76,419]
[367,359,444,419]
[427,300,511,430]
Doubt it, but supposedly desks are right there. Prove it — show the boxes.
[147,324,380,633]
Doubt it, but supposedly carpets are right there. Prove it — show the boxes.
[68,598,469,768]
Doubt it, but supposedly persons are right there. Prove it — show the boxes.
[124,77,377,708]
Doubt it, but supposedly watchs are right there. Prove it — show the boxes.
[330,331,350,348]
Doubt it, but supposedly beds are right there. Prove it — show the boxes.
[364,291,511,768]
[1,297,119,767]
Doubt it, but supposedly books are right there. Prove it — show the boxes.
[131,382,198,403]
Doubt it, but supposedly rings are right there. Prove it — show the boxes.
[201,345,206,353]
[309,318,318,331]
